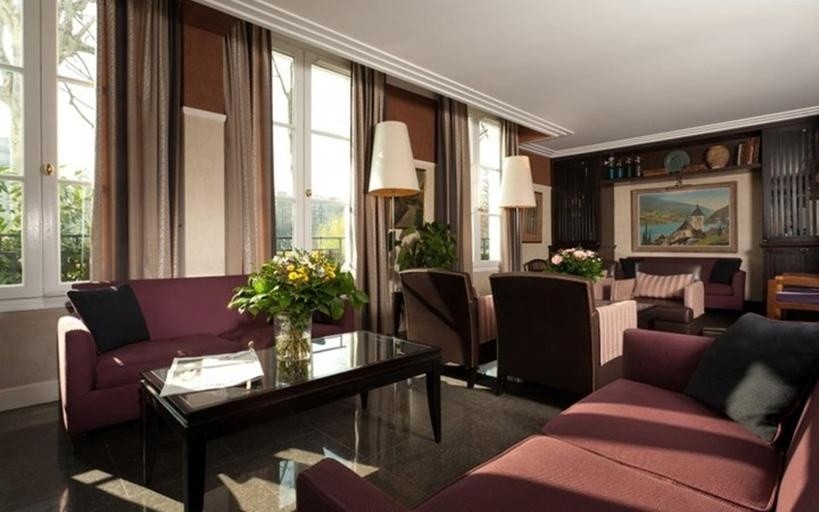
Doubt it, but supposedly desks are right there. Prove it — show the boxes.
[138,326,443,512]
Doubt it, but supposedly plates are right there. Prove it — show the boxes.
[664,149,691,175]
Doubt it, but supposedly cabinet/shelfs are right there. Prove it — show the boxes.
[765,244,819,294]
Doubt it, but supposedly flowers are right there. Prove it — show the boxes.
[547,248,604,280]
[229,249,369,362]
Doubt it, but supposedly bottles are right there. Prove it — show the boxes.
[615,157,623,179]
[623,157,632,178]
[607,156,614,180]
[634,155,641,177]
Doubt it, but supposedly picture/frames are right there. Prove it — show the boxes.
[631,182,738,253]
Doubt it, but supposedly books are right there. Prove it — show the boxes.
[735,138,761,165]
[606,155,643,179]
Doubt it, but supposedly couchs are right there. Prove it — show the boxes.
[297,310,819,512]
[489,273,638,401]
[57,273,369,438]
[398,268,497,390]
[611,262,705,336]
[611,258,749,311]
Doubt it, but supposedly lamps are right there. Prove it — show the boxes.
[500,156,536,270]
[368,121,420,267]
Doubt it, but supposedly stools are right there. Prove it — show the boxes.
[766,273,819,321]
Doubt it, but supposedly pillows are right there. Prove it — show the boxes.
[685,312,819,444]
[66,283,148,353]
